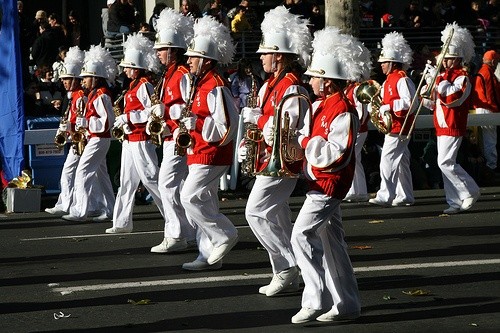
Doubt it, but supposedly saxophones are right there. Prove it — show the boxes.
[54,100,72,152]
[72,98,88,156]
[241,74,270,180]
[170,62,209,151]
[111,89,128,144]
[150,70,168,146]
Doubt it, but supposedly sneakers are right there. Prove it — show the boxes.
[443,190,480,214]
[368,198,415,207]
[44,207,361,323]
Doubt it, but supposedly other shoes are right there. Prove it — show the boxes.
[341,195,367,203]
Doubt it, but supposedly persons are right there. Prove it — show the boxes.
[106,32,160,235]
[0,161,14,215]
[63,46,115,222]
[146,9,199,256]
[239,5,308,298]
[265,28,373,326]
[420,22,480,213]
[258,0,374,204]
[356,0,500,113]
[44,49,85,215]
[176,13,237,271]
[368,32,419,208]
[16,0,264,198]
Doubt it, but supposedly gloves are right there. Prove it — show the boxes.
[263,121,275,145]
[74,117,89,132]
[56,120,72,137]
[237,145,248,164]
[179,116,198,130]
[379,104,390,117]
[113,113,132,134]
[242,106,262,125]
[367,102,373,114]
[150,100,166,118]
[424,63,441,85]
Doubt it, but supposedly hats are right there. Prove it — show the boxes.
[303,26,372,83]
[35,10,46,20]
[484,50,500,62]
[377,31,412,70]
[256,5,312,67]
[435,21,476,64]
[59,6,235,77]
[107,0,115,6]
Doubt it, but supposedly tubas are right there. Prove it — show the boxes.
[253,92,314,184]
[356,79,392,135]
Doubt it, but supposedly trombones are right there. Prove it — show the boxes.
[398,28,455,143]
